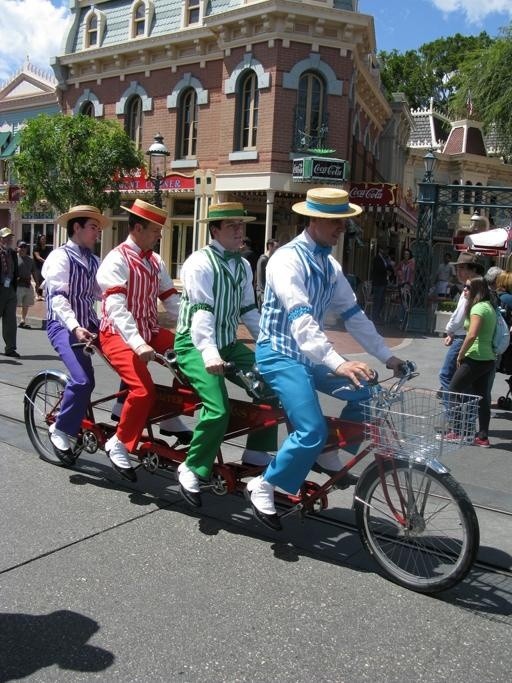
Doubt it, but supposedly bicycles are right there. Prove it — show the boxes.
[21,332,483,594]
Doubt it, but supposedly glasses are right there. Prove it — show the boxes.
[464,284,472,291]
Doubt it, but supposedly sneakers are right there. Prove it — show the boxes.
[471,436,491,448]
[436,430,466,445]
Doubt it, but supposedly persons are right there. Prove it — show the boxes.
[244,187,412,532]
[238,237,258,310]
[433,251,485,433]
[433,277,497,448]
[39,205,110,467]
[395,249,415,324]
[495,273,512,330]
[172,202,279,507]
[485,266,504,309]
[0,227,20,358]
[432,254,457,311]
[33,234,51,301]
[94,198,194,482]
[257,239,279,315]
[16,241,40,330]
[370,244,396,326]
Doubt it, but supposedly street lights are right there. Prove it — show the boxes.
[146,132,170,208]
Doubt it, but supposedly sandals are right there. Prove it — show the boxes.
[18,321,32,329]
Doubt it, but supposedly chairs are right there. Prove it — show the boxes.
[398,282,428,333]
[362,280,379,322]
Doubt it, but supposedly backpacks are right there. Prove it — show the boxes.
[492,309,510,357]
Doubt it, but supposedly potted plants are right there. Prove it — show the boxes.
[434,302,466,339]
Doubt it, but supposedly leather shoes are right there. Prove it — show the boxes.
[161,428,194,442]
[48,425,76,466]
[104,442,138,483]
[176,464,203,508]
[244,482,283,532]
[313,458,364,484]
[241,454,277,468]
[5,350,20,358]
[111,412,121,420]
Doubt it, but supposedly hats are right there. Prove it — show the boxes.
[291,188,363,218]
[17,240,29,248]
[448,252,485,274]
[55,204,111,228]
[0,227,16,239]
[195,203,256,224]
[120,199,170,230]
